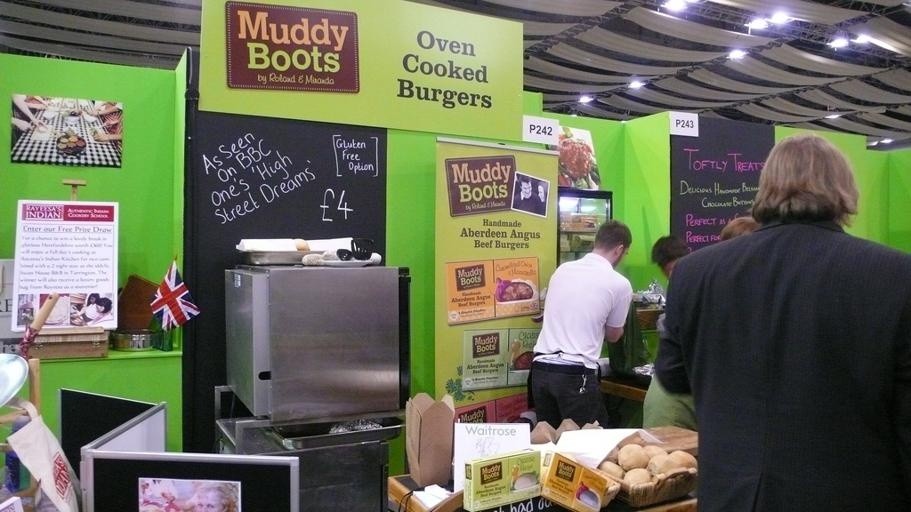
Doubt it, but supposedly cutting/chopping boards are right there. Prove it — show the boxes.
[633,426,700,454]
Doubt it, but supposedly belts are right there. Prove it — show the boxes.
[532,361,595,374]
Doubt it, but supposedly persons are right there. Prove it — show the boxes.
[641,214,762,430]
[650,234,690,281]
[512,175,540,213]
[528,221,636,426]
[70,293,100,322]
[11,94,49,133]
[642,136,911,510]
[79,297,113,326]
[88,101,123,143]
[169,480,240,511]
[534,180,549,217]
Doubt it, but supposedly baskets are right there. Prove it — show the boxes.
[594,467,699,508]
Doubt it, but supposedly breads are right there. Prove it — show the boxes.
[600,445,699,485]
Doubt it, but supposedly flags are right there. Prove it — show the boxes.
[150,255,200,332]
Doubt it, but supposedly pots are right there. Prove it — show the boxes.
[112,330,162,350]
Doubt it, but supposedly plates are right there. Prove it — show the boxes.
[324,260,372,266]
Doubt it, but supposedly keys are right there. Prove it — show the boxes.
[579,386,586,393]
